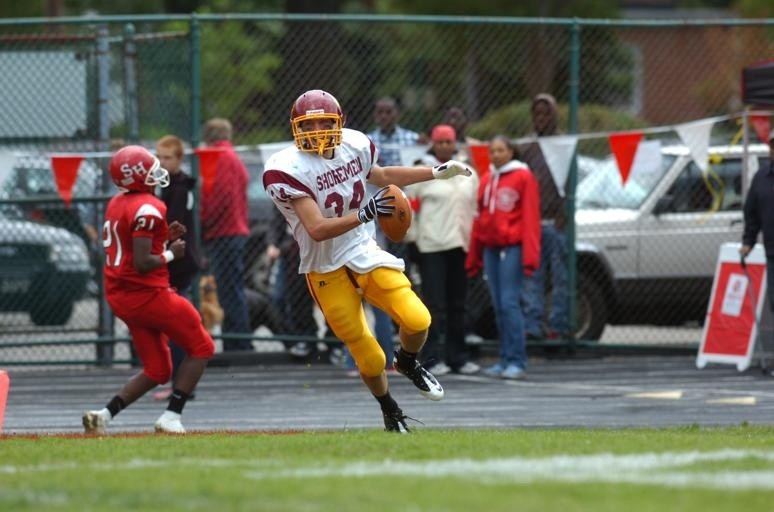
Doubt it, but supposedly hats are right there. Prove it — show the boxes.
[431,124,456,142]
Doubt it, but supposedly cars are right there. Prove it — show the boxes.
[0,215,92,329]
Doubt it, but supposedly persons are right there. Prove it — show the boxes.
[260,86,473,436]
[266,93,573,380]
[737,128,774,316]
[78,117,254,353]
[81,140,217,437]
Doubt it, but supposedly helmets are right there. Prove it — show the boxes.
[110,145,160,193]
[289,89,344,129]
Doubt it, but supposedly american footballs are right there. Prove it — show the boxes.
[377,185,411,243]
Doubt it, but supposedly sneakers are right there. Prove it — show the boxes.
[82,410,106,435]
[465,333,484,345]
[380,399,410,434]
[392,348,444,401]
[502,365,527,379]
[459,362,480,374]
[154,410,187,434]
[485,364,504,378]
[428,360,452,376]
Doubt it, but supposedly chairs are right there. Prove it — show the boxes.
[687,179,715,212]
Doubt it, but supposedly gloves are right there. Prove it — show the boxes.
[357,186,396,224]
[432,159,472,179]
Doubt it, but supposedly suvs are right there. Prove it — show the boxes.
[567,143,774,343]
